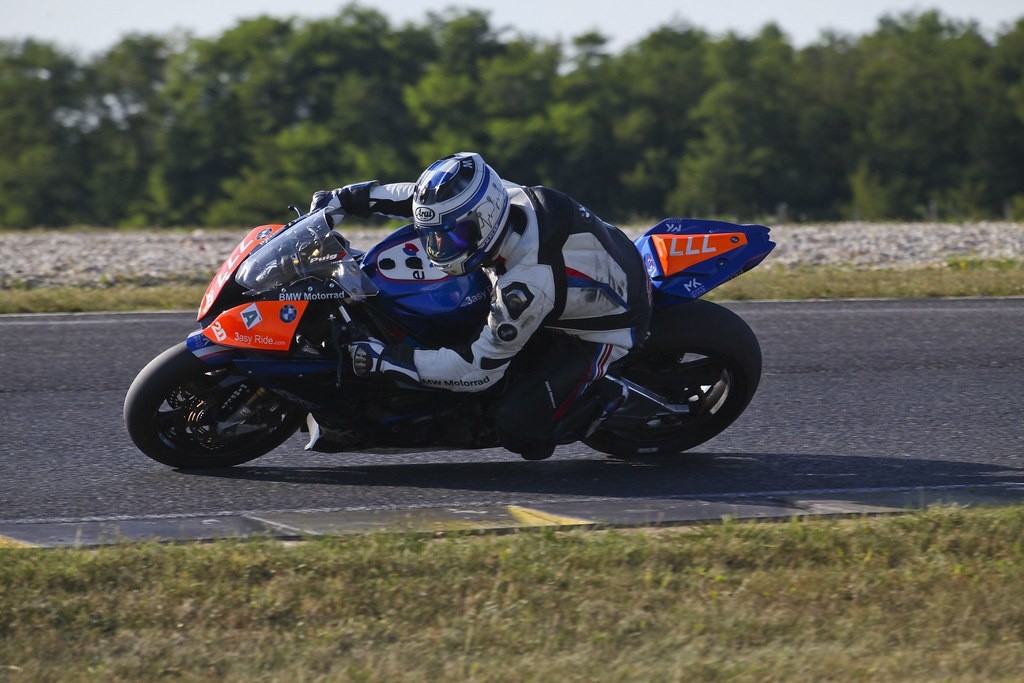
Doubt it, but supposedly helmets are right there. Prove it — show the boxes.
[414,151,512,276]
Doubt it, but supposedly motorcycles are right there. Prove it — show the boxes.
[122,203,776,471]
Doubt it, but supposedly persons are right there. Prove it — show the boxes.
[309,151,651,460]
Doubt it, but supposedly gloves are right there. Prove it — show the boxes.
[310,180,381,230]
[348,337,422,386]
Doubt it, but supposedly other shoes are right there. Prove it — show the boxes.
[576,380,630,438]
